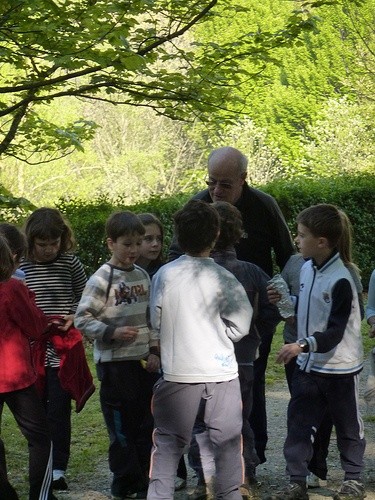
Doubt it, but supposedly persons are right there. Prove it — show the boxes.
[0,148,375,500]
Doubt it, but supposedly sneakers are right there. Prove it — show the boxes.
[267,484,309,500]
[306,472,328,488]
[333,480,367,500]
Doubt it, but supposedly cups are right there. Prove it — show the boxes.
[11,268,25,283]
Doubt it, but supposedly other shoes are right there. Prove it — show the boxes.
[111,482,146,499]
[53,477,69,493]
[187,476,205,499]
[174,477,186,491]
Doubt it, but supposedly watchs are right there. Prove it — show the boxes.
[296,338,309,353]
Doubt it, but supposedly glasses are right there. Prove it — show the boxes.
[206,173,243,189]
